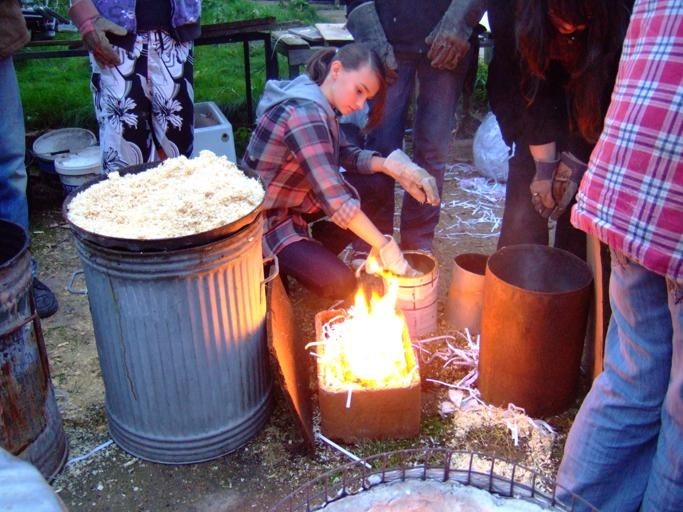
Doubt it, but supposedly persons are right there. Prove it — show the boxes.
[543,0,682,509]
[482,1,638,265]
[1,2,62,319]
[68,2,205,174]
[342,2,493,267]
[231,42,444,302]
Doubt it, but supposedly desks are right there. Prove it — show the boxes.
[268,22,356,80]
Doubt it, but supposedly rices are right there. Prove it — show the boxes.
[66,149,266,240]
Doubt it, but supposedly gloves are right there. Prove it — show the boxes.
[530,150,589,222]
[529,152,560,209]
[424,0,489,75]
[381,148,443,208]
[345,1,401,87]
[364,232,425,282]
[66,0,128,70]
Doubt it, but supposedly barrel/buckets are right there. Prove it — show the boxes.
[32,127,96,174]
[54,146,104,193]
[0,219,68,486]
[385,251,438,338]
[478,244,592,417]
[443,254,484,336]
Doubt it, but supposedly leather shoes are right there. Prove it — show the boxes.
[32,276,59,320]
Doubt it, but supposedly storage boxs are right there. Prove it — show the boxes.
[193,101,239,171]
